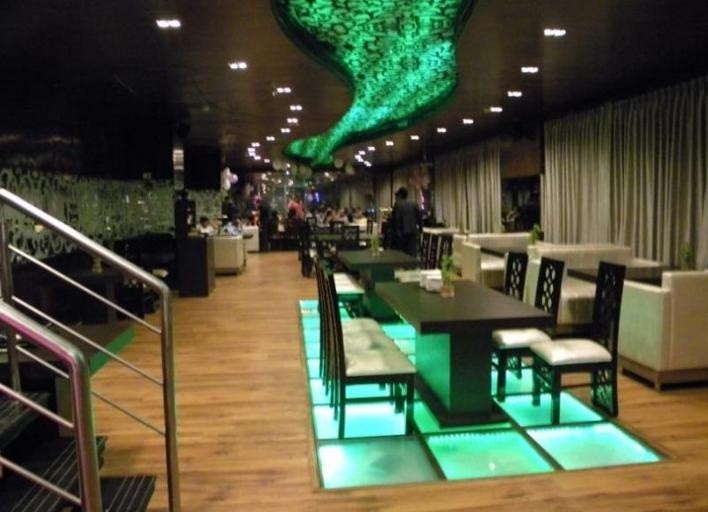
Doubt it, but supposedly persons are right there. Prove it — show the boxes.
[316,199,362,225]
[256,195,309,227]
[507,206,519,222]
[390,186,419,254]
[190,212,243,235]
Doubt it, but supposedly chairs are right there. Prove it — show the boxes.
[461,241,481,284]
[313,254,382,386]
[429,234,439,268]
[451,234,467,270]
[318,259,400,408]
[504,251,529,302]
[341,226,360,250]
[492,257,565,403]
[422,233,430,265]
[437,234,453,269]
[323,268,417,439]
[531,261,627,428]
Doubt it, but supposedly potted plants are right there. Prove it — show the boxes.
[371,232,380,257]
[528,224,541,245]
[441,255,455,299]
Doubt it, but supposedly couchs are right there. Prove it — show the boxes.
[468,232,535,288]
[528,246,635,333]
[619,269,707,391]
[215,234,244,275]
[523,244,621,259]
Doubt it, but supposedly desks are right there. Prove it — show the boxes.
[374,277,554,416]
[338,248,418,319]
[480,246,527,259]
[567,266,675,284]
